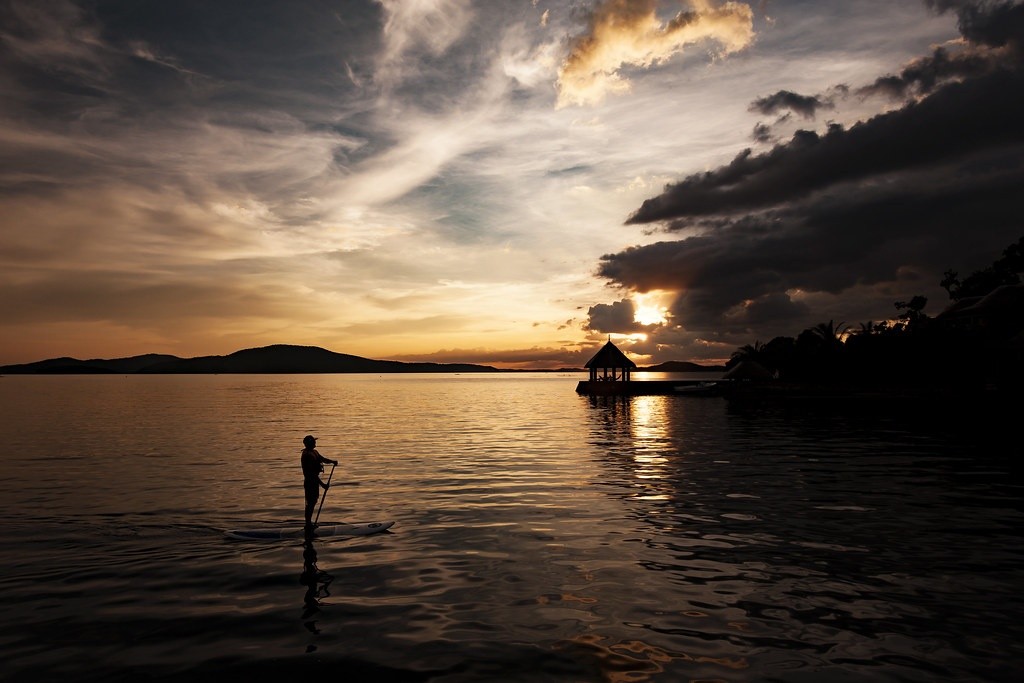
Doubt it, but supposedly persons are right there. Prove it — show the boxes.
[301,435,338,533]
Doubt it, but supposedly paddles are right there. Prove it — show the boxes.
[314,464,335,522]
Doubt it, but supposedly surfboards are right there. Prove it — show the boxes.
[233,521,394,536]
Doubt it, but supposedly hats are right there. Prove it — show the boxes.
[303,435,318,444]
